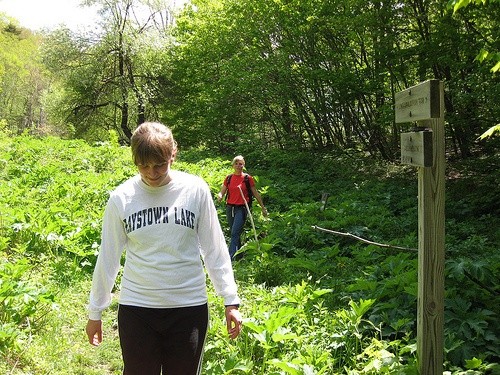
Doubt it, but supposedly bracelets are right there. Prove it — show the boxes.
[224,303,240,308]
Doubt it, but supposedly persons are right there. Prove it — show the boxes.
[85,118,242,374]
[216,154,269,260]
